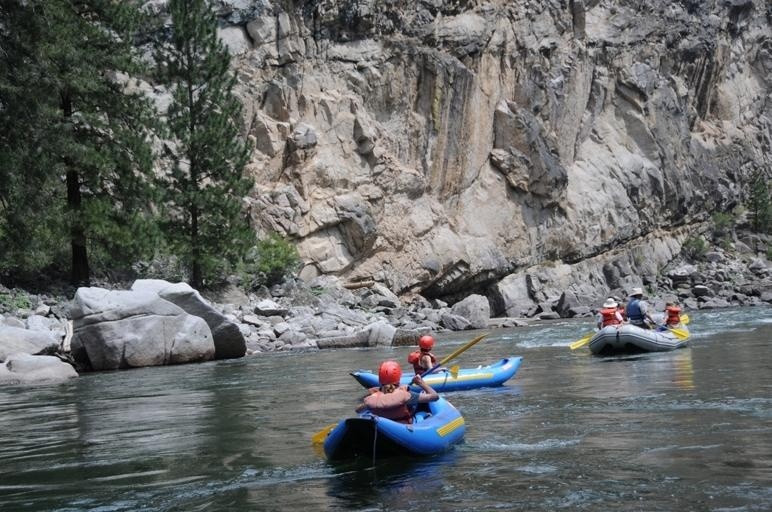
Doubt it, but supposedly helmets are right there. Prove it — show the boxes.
[380,362,401,383]
[420,336,433,349]
[407,351,421,364]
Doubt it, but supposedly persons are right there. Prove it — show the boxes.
[597,288,681,332]
[414,336,448,375]
[363,360,439,424]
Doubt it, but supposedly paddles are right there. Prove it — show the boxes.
[680,314,690,324]
[312,334,489,444]
[570,334,595,350]
[442,365,459,379]
[643,317,689,341]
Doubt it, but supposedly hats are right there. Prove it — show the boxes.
[629,289,643,295]
[603,298,618,307]
[666,299,673,304]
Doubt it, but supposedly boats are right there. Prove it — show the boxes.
[322,386,468,457]
[347,356,523,393]
[588,312,691,352]
